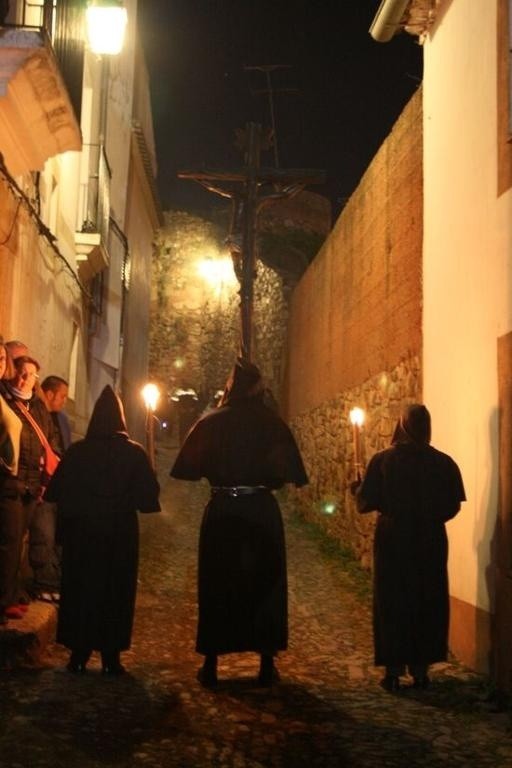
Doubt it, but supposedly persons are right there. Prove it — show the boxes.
[41,383,163,681]
[170,357,311,691]
[0,333,75,619]
[349,401,469,692]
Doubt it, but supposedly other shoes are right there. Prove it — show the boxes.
[379,677,399,692]
[258,666,278,683]
[198,666,218,687]
[65,661,85,674]
[414,673,431,688]
[103,663,123,678]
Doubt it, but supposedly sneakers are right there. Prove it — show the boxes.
[2,602,29,620]
[38,590,60,601]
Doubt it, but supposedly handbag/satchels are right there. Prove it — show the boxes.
[38,443,61,487]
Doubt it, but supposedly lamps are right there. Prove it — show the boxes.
[86,0,127,54]
